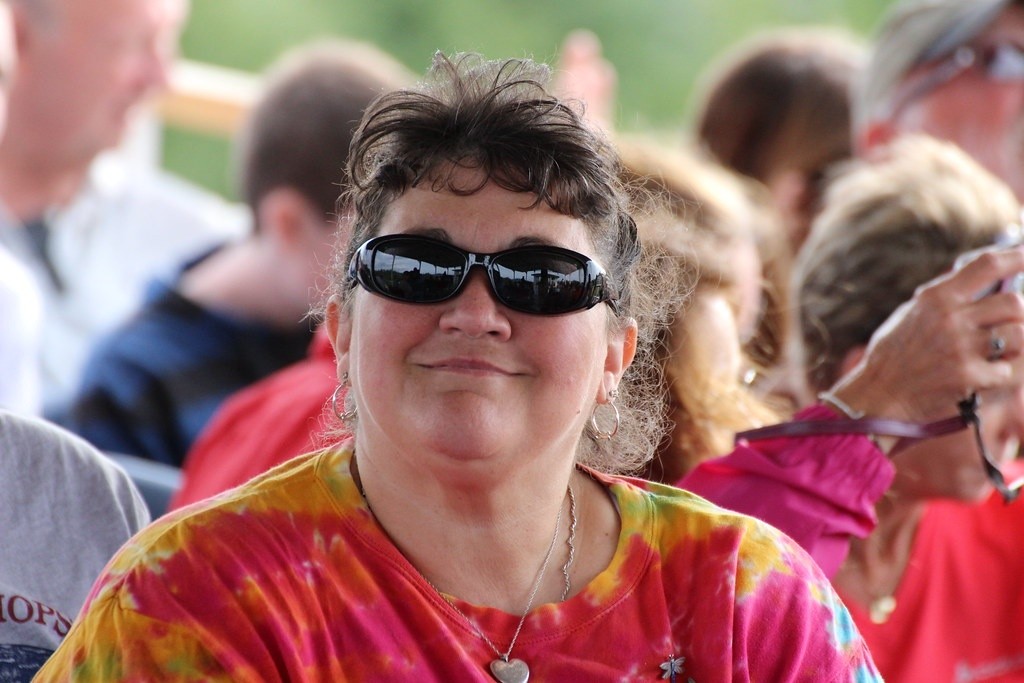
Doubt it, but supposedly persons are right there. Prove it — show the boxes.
[0,0,1024,683]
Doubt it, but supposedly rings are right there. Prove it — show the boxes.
[990,328,1004,360]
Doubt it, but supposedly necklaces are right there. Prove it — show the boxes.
[362,480,577,683]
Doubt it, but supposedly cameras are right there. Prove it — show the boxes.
[951,242,1024,304]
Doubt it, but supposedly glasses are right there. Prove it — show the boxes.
[889,42,1024,122]
[344,233,623,317]
